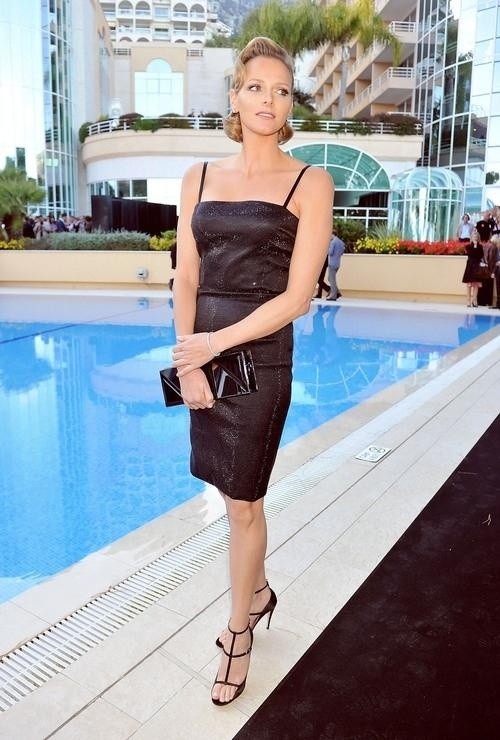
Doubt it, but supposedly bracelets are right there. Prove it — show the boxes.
[207,332,220,356]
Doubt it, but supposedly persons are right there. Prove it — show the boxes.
[23,213,92,239]
[168,233,176,290]
[312,255,342,299]
[457,205,500,310]
[326,230,345,301]
[171,37,335,707]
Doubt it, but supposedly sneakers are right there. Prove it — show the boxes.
[312,288,341,300]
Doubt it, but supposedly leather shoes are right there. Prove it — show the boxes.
[467,301,478,307]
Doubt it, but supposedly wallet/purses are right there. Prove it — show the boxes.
[160,350,259,407]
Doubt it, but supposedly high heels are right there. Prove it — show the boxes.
[216,581,277,648]
[211,618,253,706]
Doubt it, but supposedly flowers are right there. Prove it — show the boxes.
[354,235,469,255]
